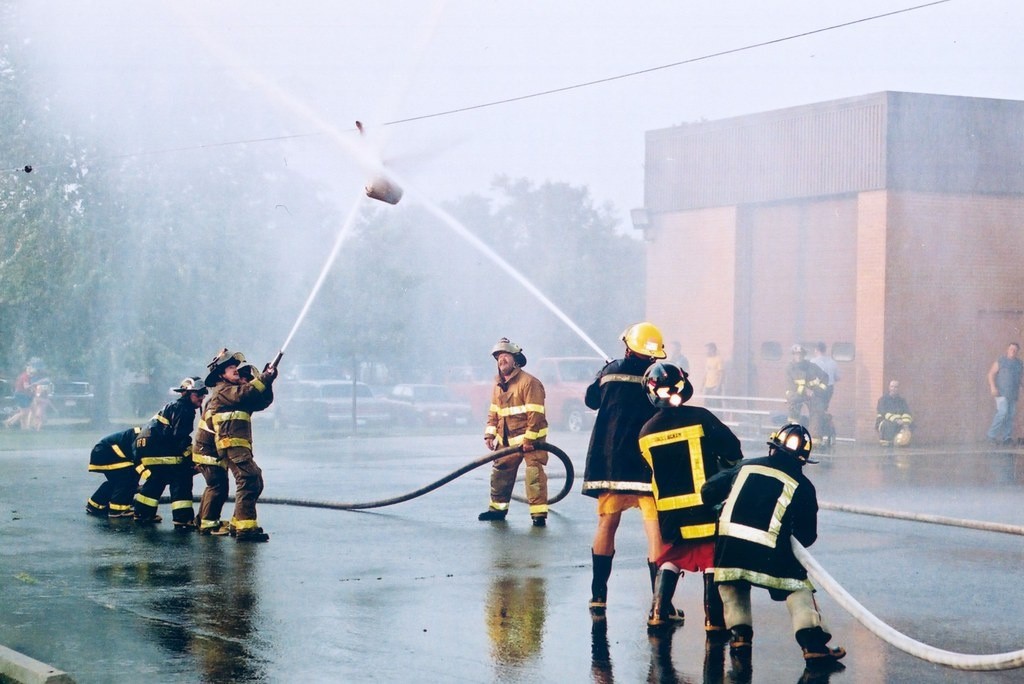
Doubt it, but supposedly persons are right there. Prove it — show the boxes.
[205,350,278,543]
[701,423,846,660]
[191,363,258,534]
[784,341,841,448]
[667,340,688,372]
[701,342,723,416]
[2,365,44,432]
[86,427,143,517]
[874,379,916,448]
[987,342,1024,447]
[637,362,743,633]
[131,375,208,531]
[478,337,548,526]
[585,323,684,622]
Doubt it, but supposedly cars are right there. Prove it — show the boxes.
[258,360,474,435]
[53,381,94,420]
[531,356,608,432]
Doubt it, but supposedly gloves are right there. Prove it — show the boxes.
[267,366,278,377]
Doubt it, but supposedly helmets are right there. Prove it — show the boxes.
[204,350,246,387]
[173,377,206,394]
[792,345,807,354]
[492,341,527,369]
[894,428,912,447]
[236,364,261,380]
[620,323,667,359]
[641,363,695,408]
[767,423,819,464]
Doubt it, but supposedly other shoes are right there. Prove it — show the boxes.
[86,509,269,541]
[730,626,754,647]
[478,510,506,521]
[801,641,846,661]
[804,659,845,675]
[731,645,752,665]
[533,517,546,527]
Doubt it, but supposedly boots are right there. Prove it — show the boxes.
[704,571,730,636]
[703,631,731,684]
[591,609,612,669]
[590,547,615,608]
[647,627,677,684]
[648,567,684,626]
[645,558,685,621]
